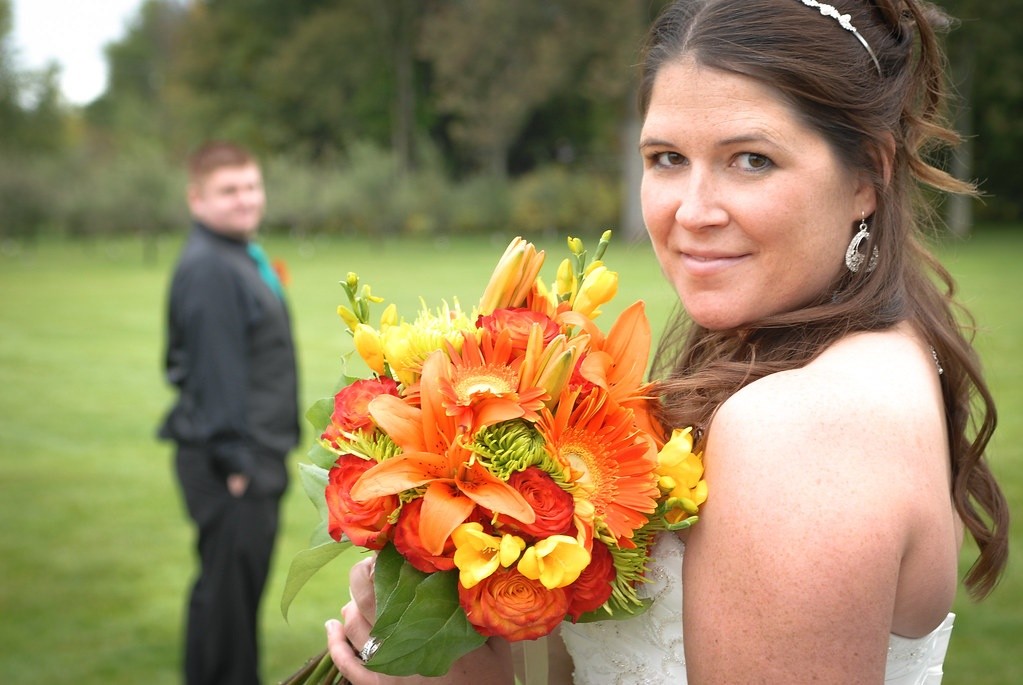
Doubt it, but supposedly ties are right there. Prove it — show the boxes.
[247,241,281,306]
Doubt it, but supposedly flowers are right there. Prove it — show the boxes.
[271,258,291,286]
[279,230,707,685]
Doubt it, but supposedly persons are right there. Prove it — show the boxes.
[322,1,963,685]
[157,144,308,685]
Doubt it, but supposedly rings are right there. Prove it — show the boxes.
[360,635,387,663]
